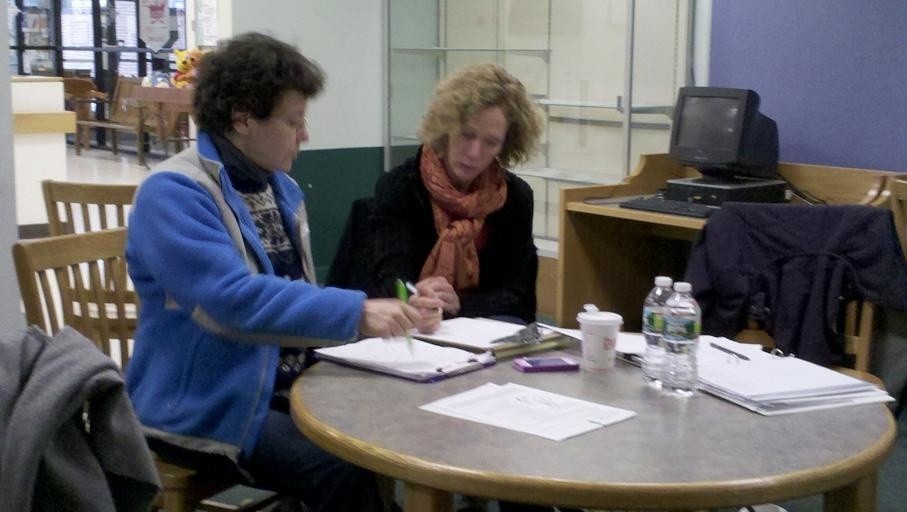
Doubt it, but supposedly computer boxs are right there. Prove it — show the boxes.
[665,177,787,211]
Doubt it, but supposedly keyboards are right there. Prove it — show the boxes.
[618,192,716,220]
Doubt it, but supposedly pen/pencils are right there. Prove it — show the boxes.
[710,342,750,361]
[397,279,414,351]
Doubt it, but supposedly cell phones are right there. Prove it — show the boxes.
[513,356,581,374]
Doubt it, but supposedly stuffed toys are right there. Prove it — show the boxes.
[169,44,204,89]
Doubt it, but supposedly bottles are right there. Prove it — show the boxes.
[662,282,700,390]
[642,277,672,378]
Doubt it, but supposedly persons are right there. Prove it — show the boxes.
[321,65,537,329]
[121,34,443,512]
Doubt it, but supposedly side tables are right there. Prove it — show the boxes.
[129,86,195,166]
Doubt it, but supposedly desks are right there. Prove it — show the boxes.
[554,150,907,371]
[293,325,895,511]
[872,174,907,265]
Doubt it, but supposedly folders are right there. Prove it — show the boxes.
[314,334,494,381]
[412,314,563,359]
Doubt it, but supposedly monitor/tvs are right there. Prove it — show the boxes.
[670,87,780,183]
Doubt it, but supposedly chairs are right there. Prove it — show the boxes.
[14,228,243,512]
[43,182,140,333]
[76,75,143,155]
[686,202,895,398]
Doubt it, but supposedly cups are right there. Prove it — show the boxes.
[577,310,623,374]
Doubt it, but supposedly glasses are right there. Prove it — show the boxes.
[242,113,311,130]
